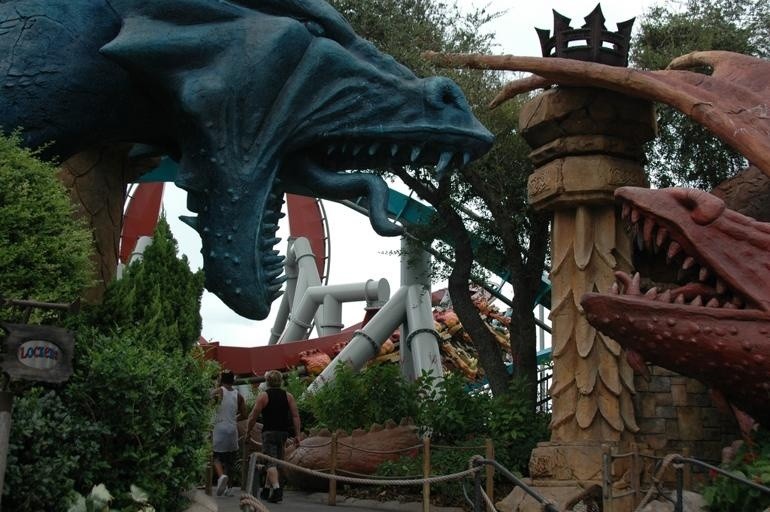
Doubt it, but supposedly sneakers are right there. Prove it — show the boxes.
[217,474,233,497]
[268,487,284,502]
[261,487,270,500]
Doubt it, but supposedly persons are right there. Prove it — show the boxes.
[242,370,301,504]
[210,370,249,497]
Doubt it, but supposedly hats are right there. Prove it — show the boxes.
[220,370,233,379]
